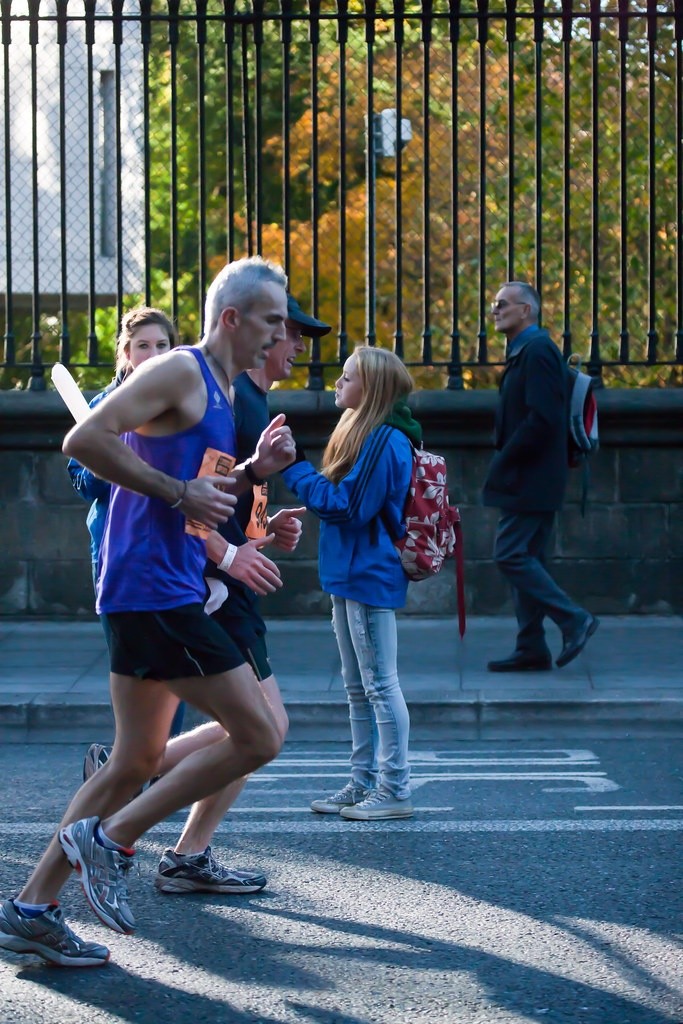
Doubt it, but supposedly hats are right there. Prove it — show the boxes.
[284,293,332,339]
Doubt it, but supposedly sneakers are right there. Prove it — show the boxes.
[0,895,110,966]
[82,743,133,811]
[310,780,372,813]
[339,785,414,819]
[57,816,135,934]
[153,845,266,894]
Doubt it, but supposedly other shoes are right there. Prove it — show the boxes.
[488,640,552,672]
[556,614,600,667]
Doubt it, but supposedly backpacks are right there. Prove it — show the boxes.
[566,353,597,468]
[377,436,461,583]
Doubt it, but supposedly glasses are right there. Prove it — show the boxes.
[490,301,525,313]
[284,324,303,340]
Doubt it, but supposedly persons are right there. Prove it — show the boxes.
[277,347,467,822]
[0,255,313,969]
[480,283,604,676]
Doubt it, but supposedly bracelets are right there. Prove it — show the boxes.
[169,480,188,509]
[216,543,238,575]
[244,457,266,488]
